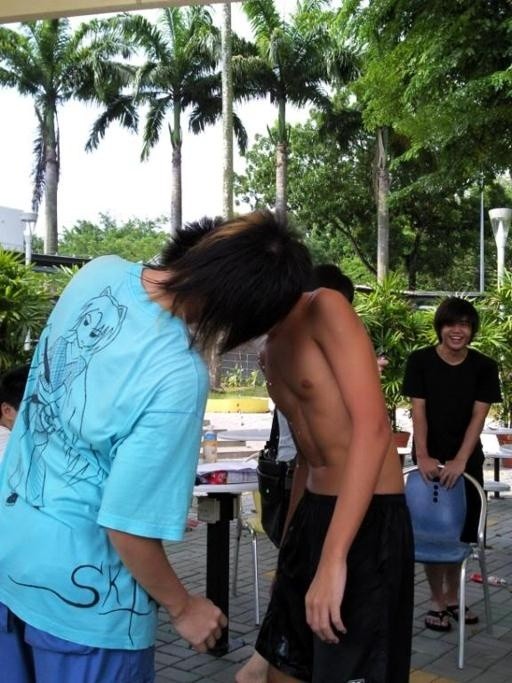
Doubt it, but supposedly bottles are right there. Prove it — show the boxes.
[203,425,217,464]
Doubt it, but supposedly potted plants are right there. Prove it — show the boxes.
[350,267,511,468]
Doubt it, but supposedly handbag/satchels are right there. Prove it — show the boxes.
[257,449,296,547]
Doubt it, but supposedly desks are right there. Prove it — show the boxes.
[190,480,261,659]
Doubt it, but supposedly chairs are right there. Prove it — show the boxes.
[401,463,493,670]
[232,451,267,626]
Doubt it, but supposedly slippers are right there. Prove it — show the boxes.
[447,604,480,624]
[425,609,453,632]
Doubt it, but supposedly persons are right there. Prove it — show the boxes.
[1,211,312,683]
[160,213,414,683]
[1,371,26,463]
[400,296,503,633]
[269,263,354,489]
[6,286,127,511]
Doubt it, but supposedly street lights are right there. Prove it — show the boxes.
[20,212,38,353]
[488,207,512,325]
